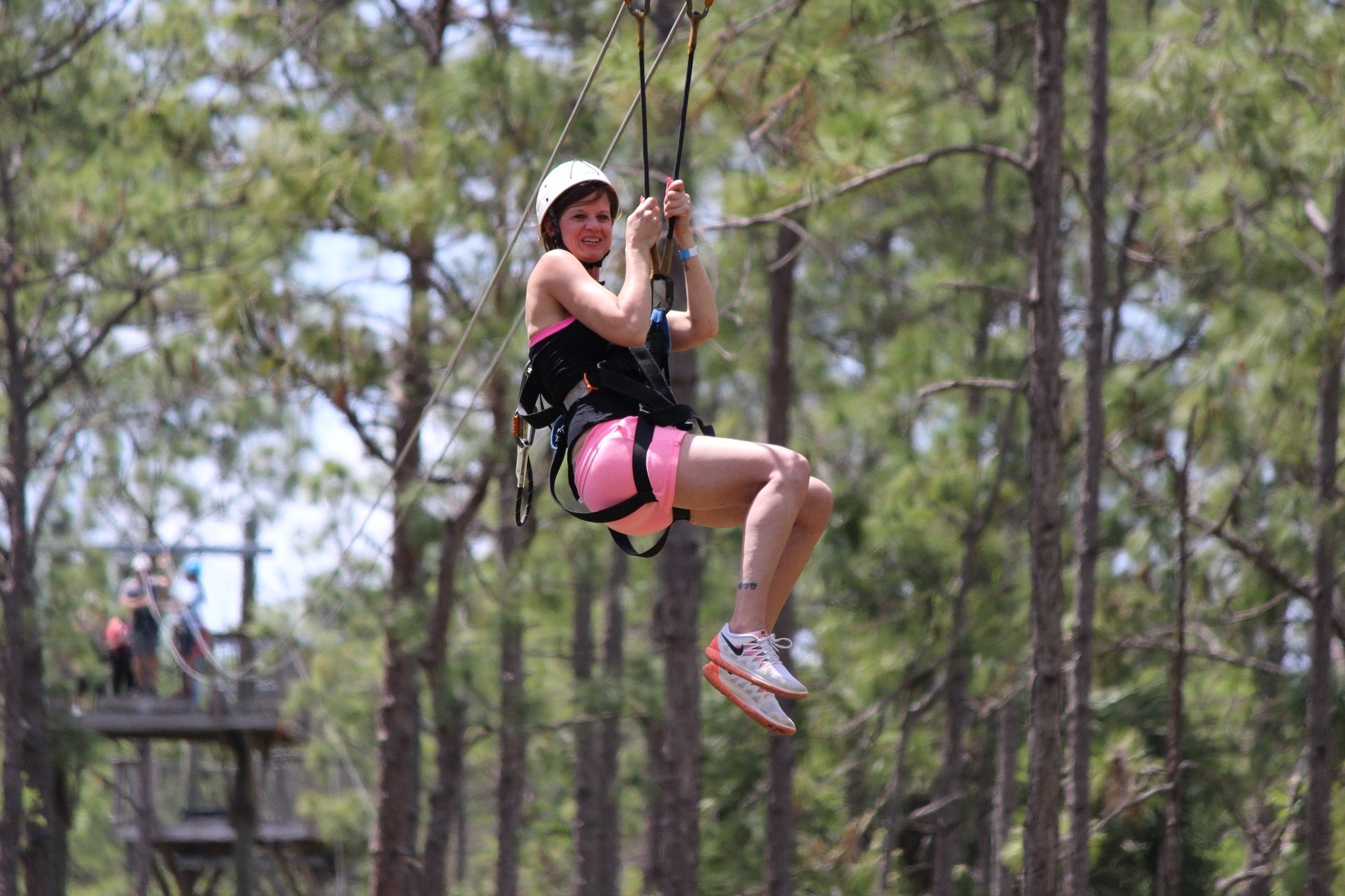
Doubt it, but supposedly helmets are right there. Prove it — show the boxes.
[131,552,153,573]
[536,159,620,238]
[181,558,200,577]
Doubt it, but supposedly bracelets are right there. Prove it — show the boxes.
[678,245,700,259]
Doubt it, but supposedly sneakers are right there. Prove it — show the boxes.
[700,658,800,735]
[700,624,811,699]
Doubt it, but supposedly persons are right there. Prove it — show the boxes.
[107,615,133,696]
[524,158,833,735]
[69,673,102,715]
[120,552,170,696]
[157,554,203,697]
